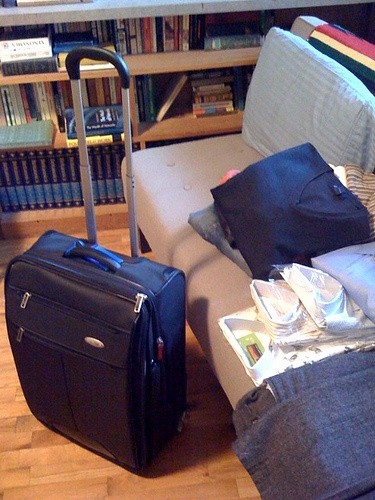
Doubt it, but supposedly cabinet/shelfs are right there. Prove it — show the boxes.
[0,0,375,239]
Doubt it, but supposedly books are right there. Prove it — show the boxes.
[203,34,265,49]
[190,67,239,117]
[1,75,162,149]
[154,75,198,122]
[0,143,134,216]
[1,9,205,78]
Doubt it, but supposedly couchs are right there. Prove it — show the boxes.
[120,16,375,499]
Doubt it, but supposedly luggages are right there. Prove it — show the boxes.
[3,46,186,475]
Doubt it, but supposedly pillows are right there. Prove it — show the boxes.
[306,23,375,95]
[240,27,375,172]
